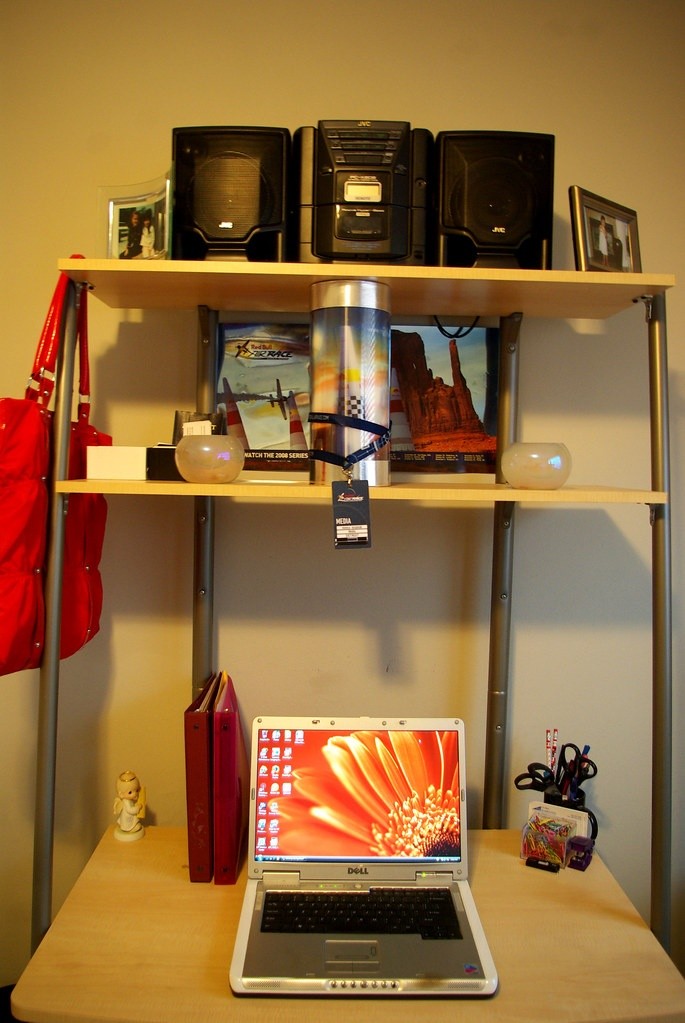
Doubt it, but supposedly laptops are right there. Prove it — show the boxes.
[228,717,498,997]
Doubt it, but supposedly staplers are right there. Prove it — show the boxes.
[567,835,596,872]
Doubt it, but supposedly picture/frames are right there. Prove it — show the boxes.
[567,186,643,276]
[100,178,173,261]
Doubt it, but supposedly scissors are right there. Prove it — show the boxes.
[514,762,561,796]
[560,743,598,800]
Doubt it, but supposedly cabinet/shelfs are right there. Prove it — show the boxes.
[30,257,683,964]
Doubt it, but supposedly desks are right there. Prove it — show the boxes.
[12,823,684,1021]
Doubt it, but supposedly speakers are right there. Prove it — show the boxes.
[167,125,295,264]
[432,130,554,270]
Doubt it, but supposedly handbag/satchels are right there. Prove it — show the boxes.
[0,254,116,676]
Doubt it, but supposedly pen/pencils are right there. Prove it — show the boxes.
[545,729,590,796]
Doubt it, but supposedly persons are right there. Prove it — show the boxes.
[139,215,155,258]
[119,211,144,259]
[114,770,146,833]
[598,215,610,266]
